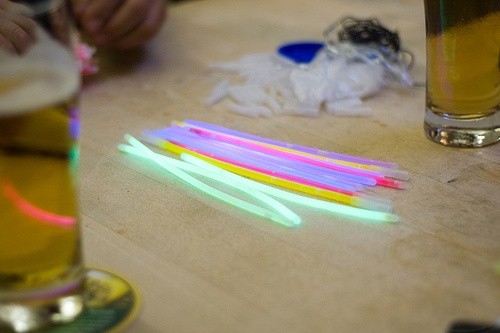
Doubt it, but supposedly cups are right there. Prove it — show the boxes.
[0,0,88,308]
[423,0,500,149]
